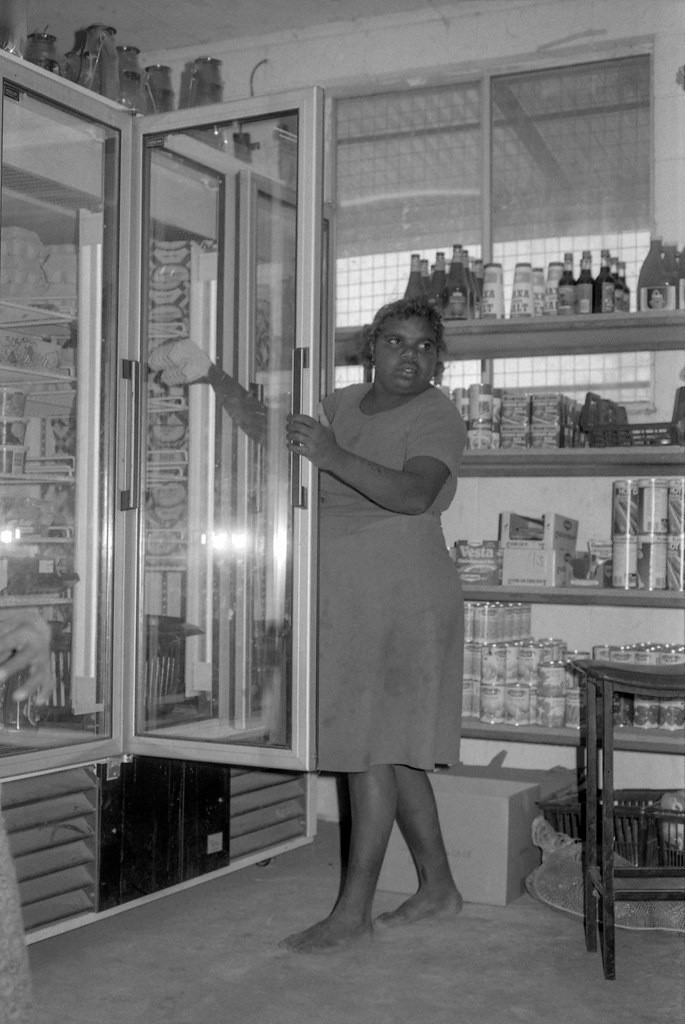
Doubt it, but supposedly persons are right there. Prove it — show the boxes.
[162,298,466,955]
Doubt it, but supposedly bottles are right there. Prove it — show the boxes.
[22,22,224,117]
[403,235,685,321]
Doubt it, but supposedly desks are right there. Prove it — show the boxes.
[571,660,685,979]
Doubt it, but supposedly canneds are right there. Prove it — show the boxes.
[439,383,506,450]
[461,603,685,732]
[610,476,685,594]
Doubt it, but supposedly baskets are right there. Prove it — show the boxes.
[645,797,685,868]
[542,789,663,869]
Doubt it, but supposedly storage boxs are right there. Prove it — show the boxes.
[36,615,187,722]
[499,512,579,549]
[456,540,502,585]
[375,772,540,906]
[500,394,586,450]
[535,788,685,868]
[502,549,575,587]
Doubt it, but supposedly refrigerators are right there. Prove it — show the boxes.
[1,52,325,946]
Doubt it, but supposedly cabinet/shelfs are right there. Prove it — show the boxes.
[332,308,685,753]
